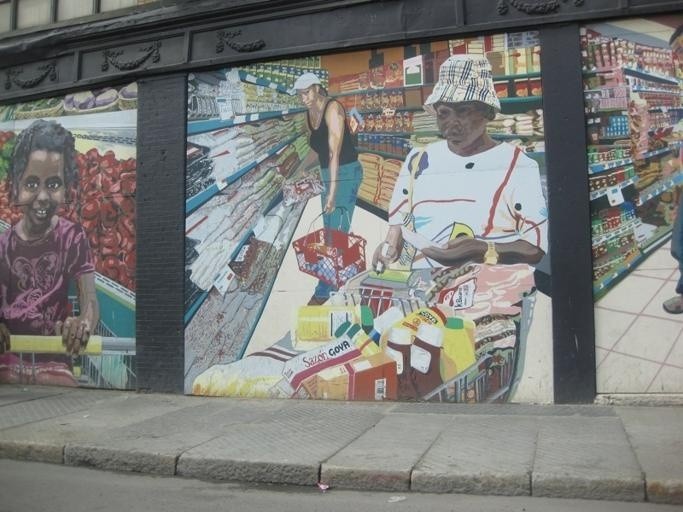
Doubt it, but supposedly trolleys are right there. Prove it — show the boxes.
[192,223,543,405]
[0,294,135,391]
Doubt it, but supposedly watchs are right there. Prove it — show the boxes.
[484,241,500,267]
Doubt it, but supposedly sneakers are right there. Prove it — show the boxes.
[663,296,683,314]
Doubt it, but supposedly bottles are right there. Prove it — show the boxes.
[332,307,445,395]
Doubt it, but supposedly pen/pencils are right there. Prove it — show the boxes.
[376,240,390,277]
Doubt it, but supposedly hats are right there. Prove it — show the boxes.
[424,54,501,113]
[288,72,321,96]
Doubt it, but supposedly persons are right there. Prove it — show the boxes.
[0,118,102,387]
[663,24,683,315]
[370,52,548,275]
[288,71,365,307]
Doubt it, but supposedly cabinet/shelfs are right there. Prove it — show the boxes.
[587,62,681,303]
[182,70,315,378]
[329,72,548,298]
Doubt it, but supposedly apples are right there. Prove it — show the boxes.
[0,131,137,293]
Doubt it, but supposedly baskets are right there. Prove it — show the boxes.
[293,207,367,287]
